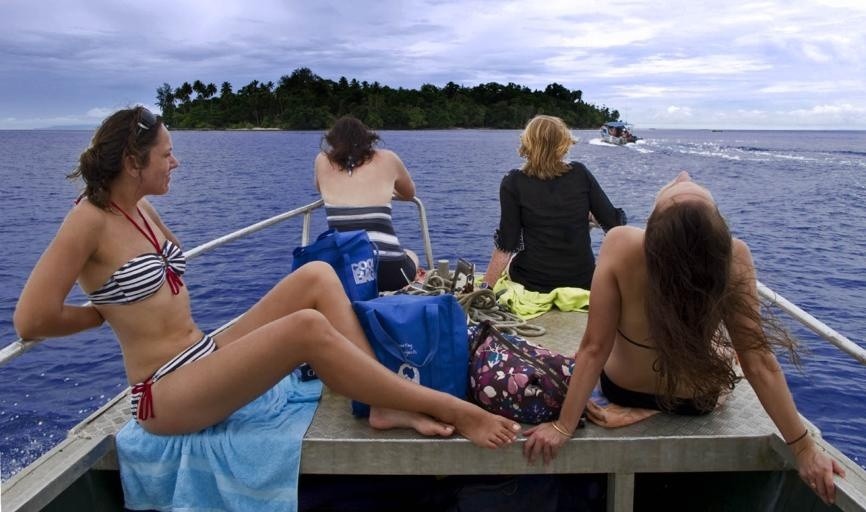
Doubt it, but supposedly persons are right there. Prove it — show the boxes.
[313,115,420,292]
[523,170,846,506]
[12,104,523,449]
[481,115,628,295]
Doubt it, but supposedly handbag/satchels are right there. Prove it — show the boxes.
[292,228,378,300]
[352,294,586,428]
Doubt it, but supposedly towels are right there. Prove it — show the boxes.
[114,367,323,511]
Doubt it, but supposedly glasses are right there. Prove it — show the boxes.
[136,108,155,136]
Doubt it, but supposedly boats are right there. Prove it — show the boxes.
[0,199,862,511]
[600,121,639,145]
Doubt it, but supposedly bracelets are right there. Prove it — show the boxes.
[550,420,573,438]
[785,428,808,446]
